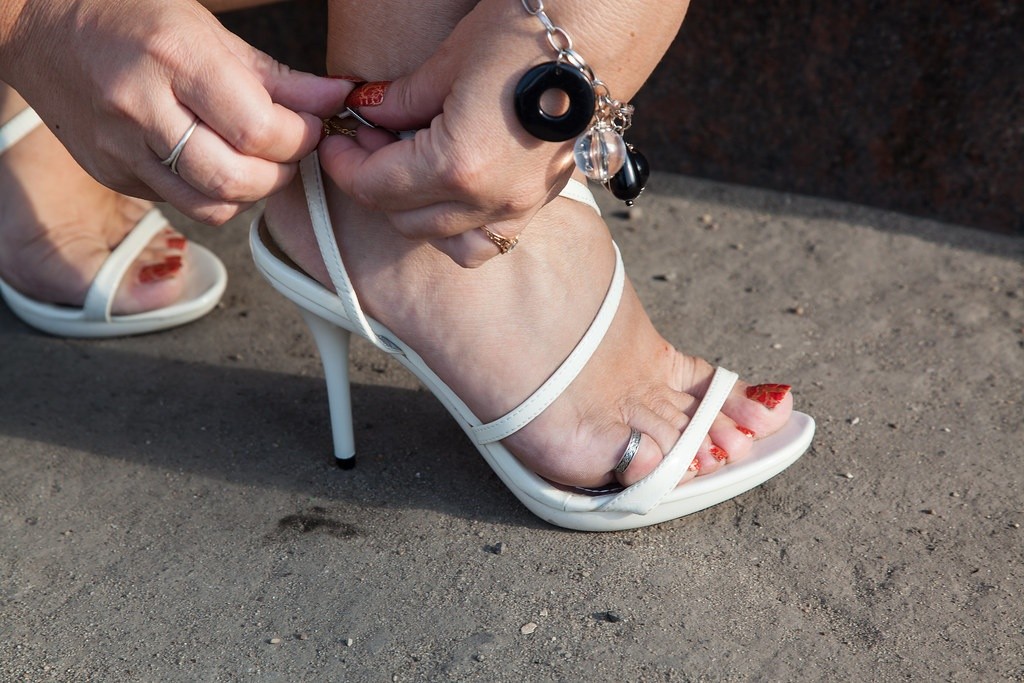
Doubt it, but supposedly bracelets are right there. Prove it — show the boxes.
[516,0,651,207]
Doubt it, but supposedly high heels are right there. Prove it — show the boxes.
[2,102,230,338]
[251,149,817,537]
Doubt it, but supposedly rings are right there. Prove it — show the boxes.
[613,425,642,471]
[477,225,519,255]
[160,114,200,176]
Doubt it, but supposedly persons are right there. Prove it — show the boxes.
[1,0,814,531]
